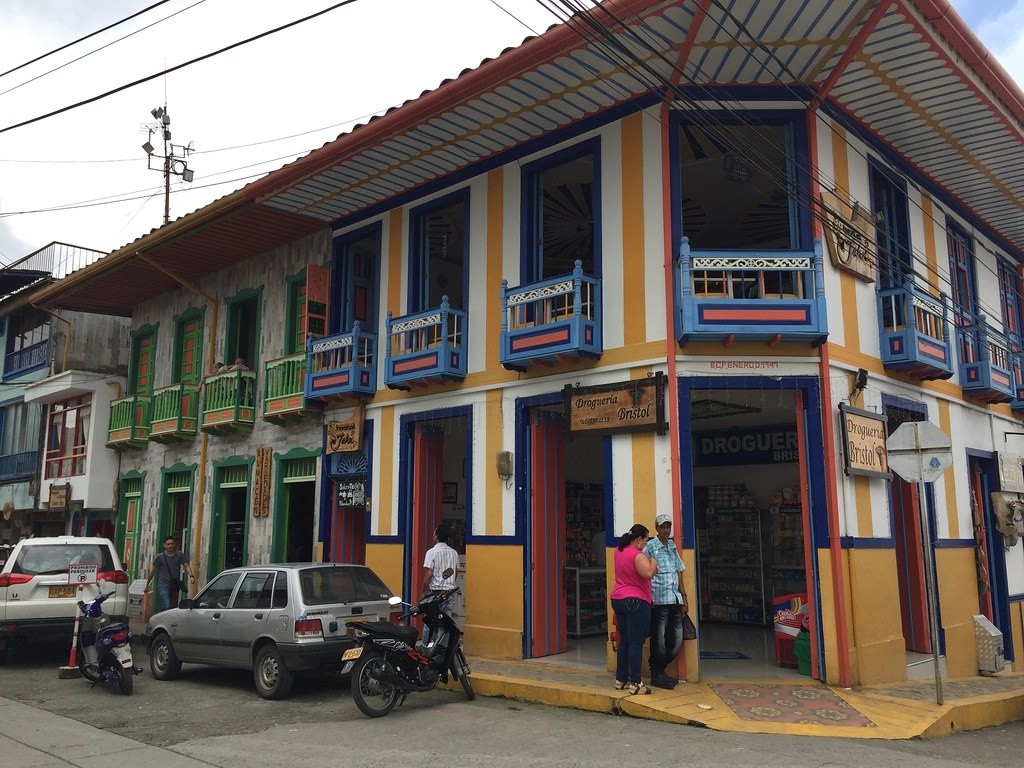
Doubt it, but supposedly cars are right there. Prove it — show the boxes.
[147,561,404,700]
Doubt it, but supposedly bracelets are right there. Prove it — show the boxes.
[682,593,686,596]
[190,575,194,578]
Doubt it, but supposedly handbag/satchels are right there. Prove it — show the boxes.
[172,577,180,592]
[683,612,696,639]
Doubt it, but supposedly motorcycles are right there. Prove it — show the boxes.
[340,568,475,717]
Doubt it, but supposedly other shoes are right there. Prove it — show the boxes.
[671,678,678,685]
[651,668,673,689]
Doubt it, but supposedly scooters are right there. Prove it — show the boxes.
[77,579,143,695]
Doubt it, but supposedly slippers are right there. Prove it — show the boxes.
[629,682,653,694]
[615,678,631,690]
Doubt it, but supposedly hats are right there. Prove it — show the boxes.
[655,514,672,526]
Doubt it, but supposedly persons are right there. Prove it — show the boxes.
[420,524,460,653]
[589,513,606,568]
[643,514,688,689]
[143,536,195,611]
[196,357,252,407]
[610,524,658,694]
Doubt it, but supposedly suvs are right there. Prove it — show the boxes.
[0,533,130,662]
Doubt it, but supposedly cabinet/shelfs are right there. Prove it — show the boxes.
[769,506,807,596]
[566,566,608,637]
[696,507,767,627]
